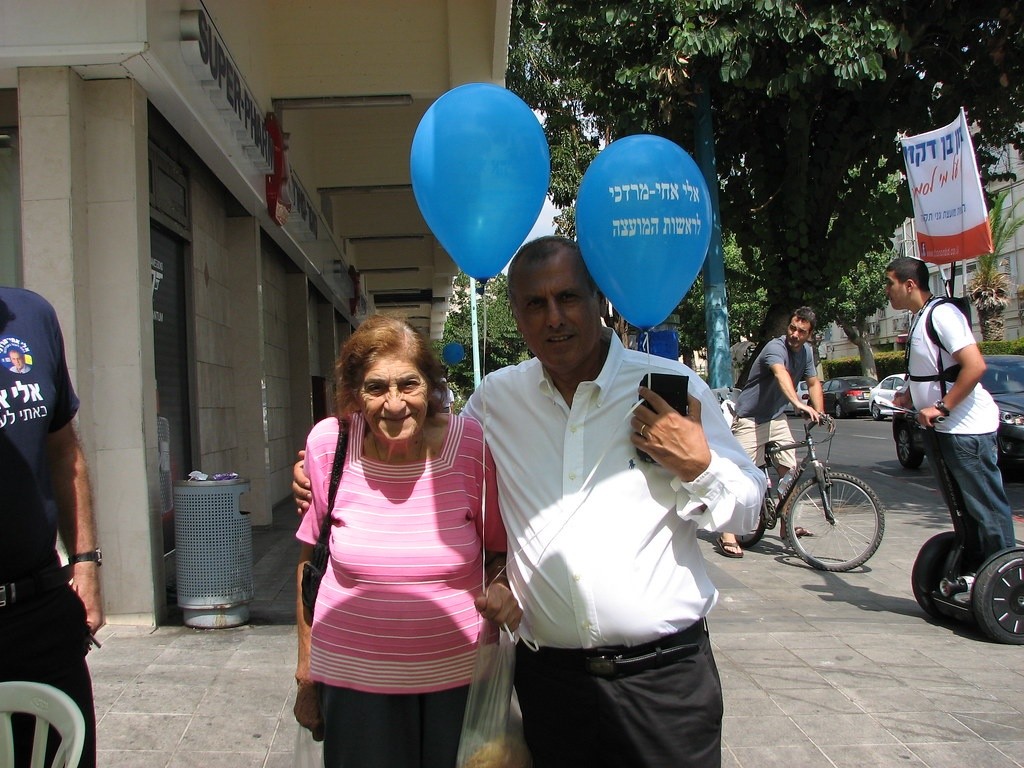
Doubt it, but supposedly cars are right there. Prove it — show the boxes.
[802,376,880,419]
[784,380,825,415]
[868,373,909,420]
[892,353,1024,480]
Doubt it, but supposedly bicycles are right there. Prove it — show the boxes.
[729,408,886,575]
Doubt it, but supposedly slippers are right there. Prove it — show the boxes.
[782,527,814,539]
[715,537,744,558]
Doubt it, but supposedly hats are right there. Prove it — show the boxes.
[440,378,447,382]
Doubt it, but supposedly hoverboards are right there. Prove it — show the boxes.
[894,408,1024,647]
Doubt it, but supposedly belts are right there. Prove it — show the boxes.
[549,620,705,675]
[0,565,73,610]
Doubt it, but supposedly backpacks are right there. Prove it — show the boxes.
[926,295,973,354]
[734,337,792,392]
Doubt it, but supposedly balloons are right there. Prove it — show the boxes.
[443,342,465,367]
[574,134,713,332]
[409,81,552,290]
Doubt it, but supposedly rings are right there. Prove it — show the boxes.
[640,424,646,436]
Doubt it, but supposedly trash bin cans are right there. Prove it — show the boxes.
[173,476,254,628]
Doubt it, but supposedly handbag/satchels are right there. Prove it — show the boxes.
[456,618,533,768]
[300,543,329,608]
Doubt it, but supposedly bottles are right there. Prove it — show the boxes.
[777,466,796,494]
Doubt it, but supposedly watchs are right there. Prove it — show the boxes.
[68,547,104,566]
[933,399,950,415]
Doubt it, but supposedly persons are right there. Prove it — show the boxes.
[8,350,31,373]
[292,314,523,768]
[0,284,108,768]
[433,377,455,415]
[885,255,1017,603]
[716,307,835,559]
[291,236,766,768]
